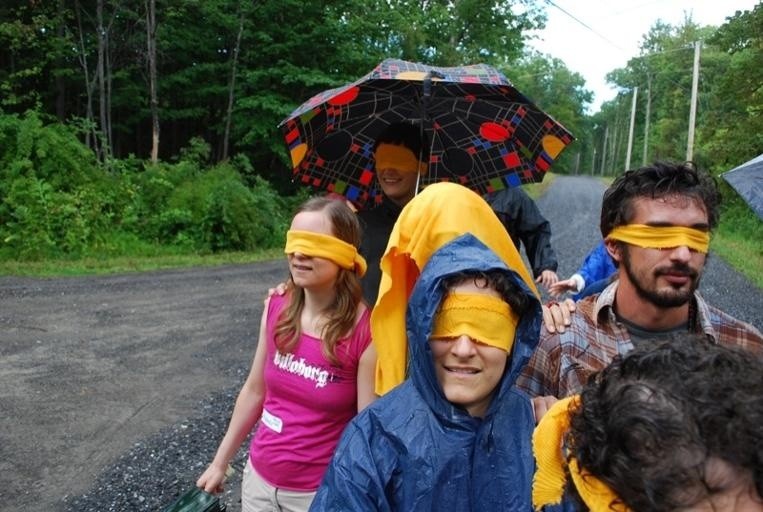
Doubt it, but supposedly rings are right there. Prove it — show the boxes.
[546,299,558,308]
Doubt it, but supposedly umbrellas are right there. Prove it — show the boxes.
[720,151,763,223]
[261,56,575,216]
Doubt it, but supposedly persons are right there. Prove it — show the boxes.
[263,119,432,312]
[529,327,761,512]
[303,233,585,511]
[194,195,380,511]
[482,186,561,290]
[369,178,578,399]
[513,158,763,426]
[544,236,618,302]
